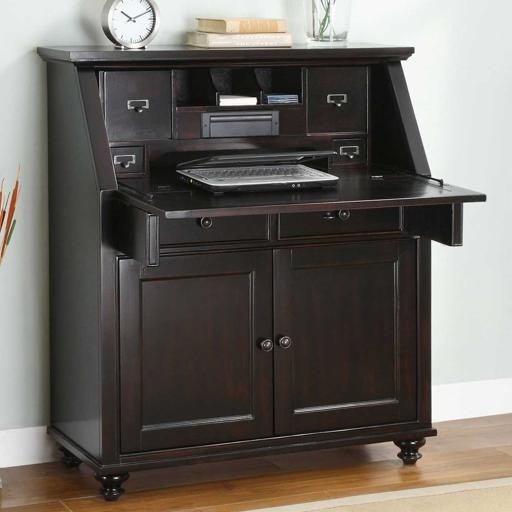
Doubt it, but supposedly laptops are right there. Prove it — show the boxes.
[177,151,340,193]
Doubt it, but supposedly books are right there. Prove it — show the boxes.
[184,32,292,48]
[216,92,259,107]
[194,17,288,34]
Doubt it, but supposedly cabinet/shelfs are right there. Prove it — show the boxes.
[36,46,486,501]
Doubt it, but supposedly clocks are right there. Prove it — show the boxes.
[100,1,163,50]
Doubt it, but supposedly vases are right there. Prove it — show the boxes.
[306,0,352,49]
